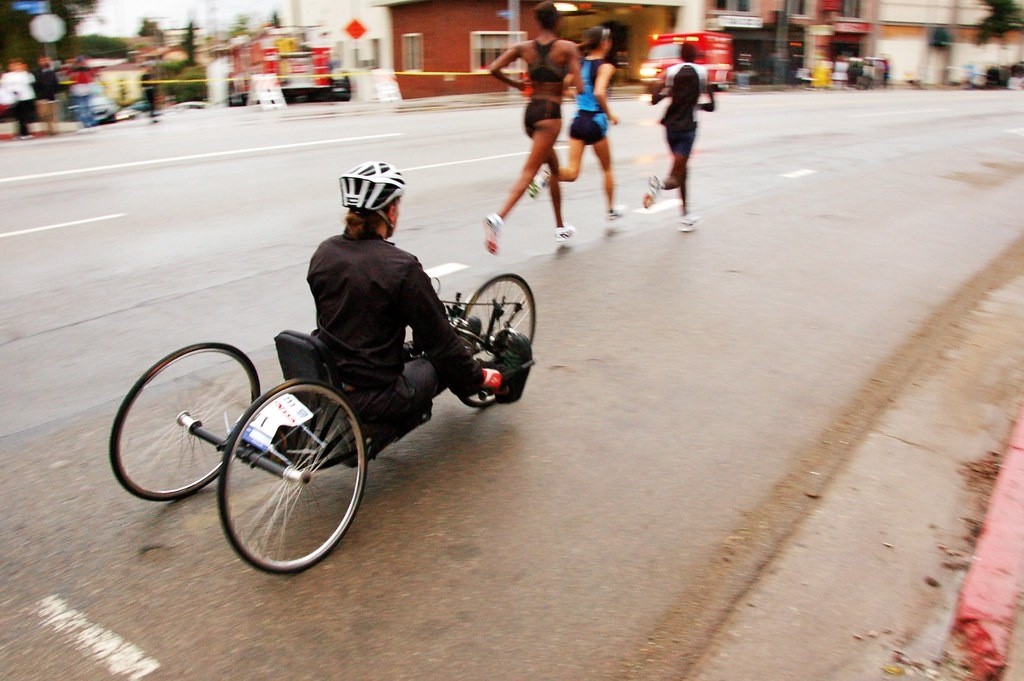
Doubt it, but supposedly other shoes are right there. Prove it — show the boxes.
[495,332,533,404]
[463,316,481,347]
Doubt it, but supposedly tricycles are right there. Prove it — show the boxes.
[109,272,538,576]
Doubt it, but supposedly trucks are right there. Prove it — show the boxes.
[640,31,734,92]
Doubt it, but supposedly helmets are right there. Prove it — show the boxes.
[338,160,406,213]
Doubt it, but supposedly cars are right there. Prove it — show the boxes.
[266,53,351,100]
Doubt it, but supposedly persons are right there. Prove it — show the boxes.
[141,64,162,117]
[736,54,751,89]
[528,27,628,221]
[483,0,584,255]
[67,57,94,132]
[643,40,715,232]
[832,54,891,90]
[306,159,502,424]
[0,54,60,142]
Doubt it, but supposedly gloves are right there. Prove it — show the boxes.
[480,367,502,395]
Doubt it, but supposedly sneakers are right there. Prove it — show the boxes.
[678,213,706,233]
[526,162,551,201]
[554,224,579,243]
[482,212,504,258]
[641,174,665,209]
[604,205,628,223]
